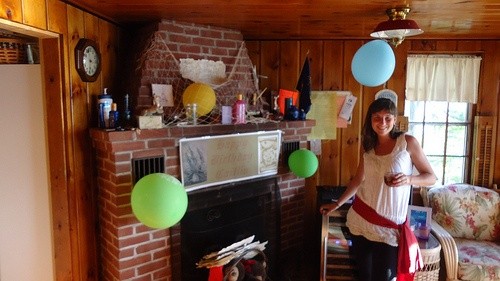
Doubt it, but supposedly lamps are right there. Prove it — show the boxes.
[369,7,425,47]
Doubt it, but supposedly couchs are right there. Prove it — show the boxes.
[421,181,500,281]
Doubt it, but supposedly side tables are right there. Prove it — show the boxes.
[411,232,442,281]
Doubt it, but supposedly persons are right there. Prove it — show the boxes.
[320,98,436,281]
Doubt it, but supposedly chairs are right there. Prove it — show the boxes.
[318,193,355,281]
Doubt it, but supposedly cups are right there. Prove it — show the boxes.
[186,103,199,125]
[384,172,398,187]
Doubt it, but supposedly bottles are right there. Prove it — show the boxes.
[97,94,112,129]
[109,102,119,129]
[232,94,247,125]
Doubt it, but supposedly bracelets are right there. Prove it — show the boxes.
[336,201,340,208]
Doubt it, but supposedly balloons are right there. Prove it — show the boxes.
[352,40,395,87]
[289,150,318,178]
[130,173,188,228]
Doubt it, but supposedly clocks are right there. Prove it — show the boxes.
[75,37,104,82]
